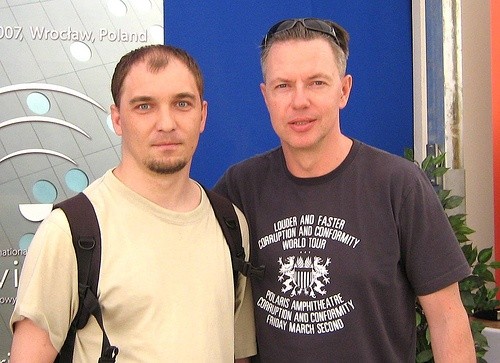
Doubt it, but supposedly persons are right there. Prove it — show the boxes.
[209,16,479,363]
[8,43,258,363]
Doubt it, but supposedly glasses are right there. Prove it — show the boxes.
[264,19,340,47]
